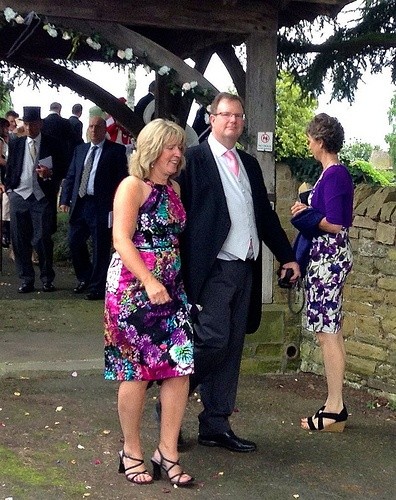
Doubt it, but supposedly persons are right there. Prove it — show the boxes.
[0,110,25,249]
[59,116,129,299]
[291,113,355,432]
[5,106,69,292]
[156,92,301,452]
[44,103,70,131]
[134,80,155,117]
[106,97,132,144]
[104,118,196,484]
[69,104,84,144]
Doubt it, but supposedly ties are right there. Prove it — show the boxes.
[30,139,37,164]
[223,151,239,177]
[78,146,99,198]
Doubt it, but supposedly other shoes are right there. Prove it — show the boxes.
[31,250,39,265]
[2,231,10,248]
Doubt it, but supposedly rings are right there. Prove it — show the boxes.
[282,267,285,270]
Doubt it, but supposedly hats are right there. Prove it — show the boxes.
[18,106,45,121]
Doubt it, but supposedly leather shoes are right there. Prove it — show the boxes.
[198,428,257,453]
[42,281,55,292]
[155,402,190,452]
[73,280,89,294]
[17,283,34,292]
[85,286,105,300]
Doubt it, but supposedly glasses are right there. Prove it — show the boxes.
[212,112,246,121]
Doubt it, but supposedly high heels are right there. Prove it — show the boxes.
[301,404,348,432]
[118,448,153,484]
[150,446,197,484]
[300,405,326,424]
